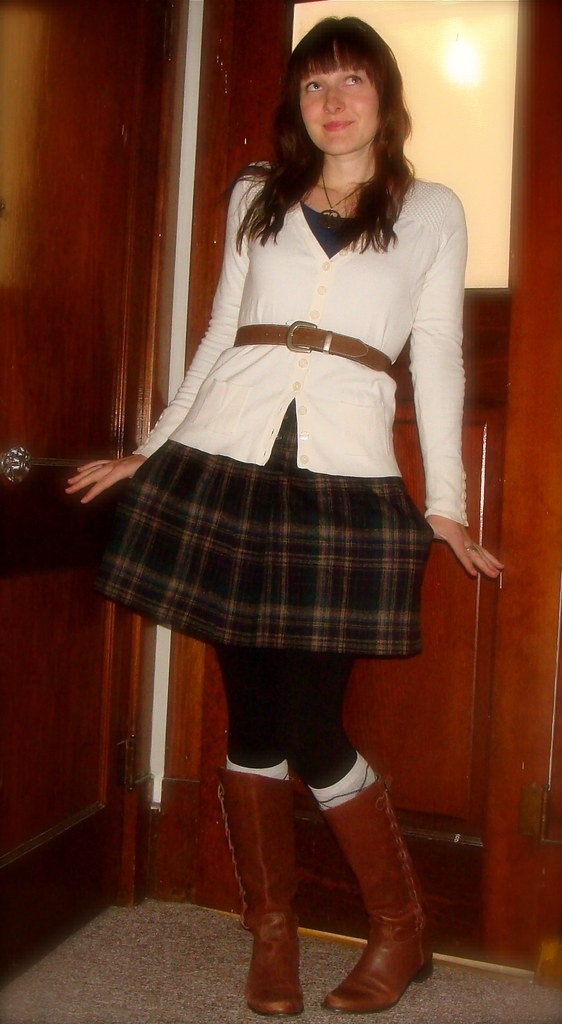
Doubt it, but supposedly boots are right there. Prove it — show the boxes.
[213,765,304,1016]
[319,773,433,1015]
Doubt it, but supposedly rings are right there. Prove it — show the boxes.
[466,545,475,551]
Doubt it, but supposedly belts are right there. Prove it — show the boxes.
[234,321,391,374]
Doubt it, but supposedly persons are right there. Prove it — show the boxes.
[66,16,504,1014]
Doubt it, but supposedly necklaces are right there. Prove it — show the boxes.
[318,167,373,229]
